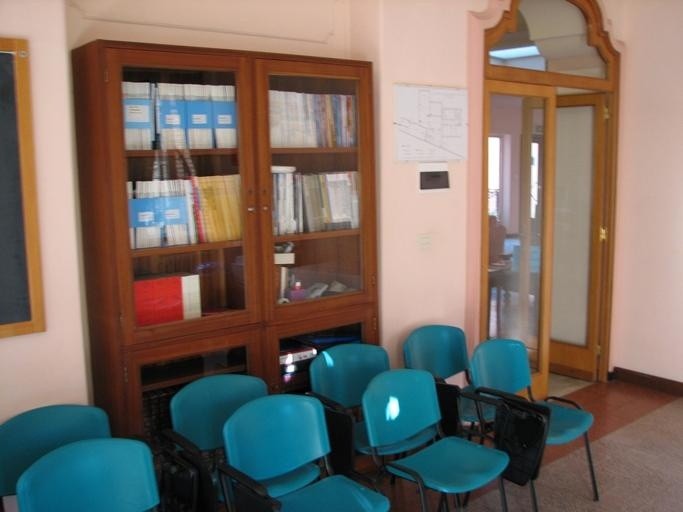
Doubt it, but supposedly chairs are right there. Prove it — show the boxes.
[215,392,391,511]
[17,438,164,512]
[0,404,111,510]
[164,374,322,511]
[362,368,547,512]
[401,327,496,442]
[469,339,599,511]
[310,342,437,488]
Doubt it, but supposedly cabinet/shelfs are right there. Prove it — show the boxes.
[70,38,381,472]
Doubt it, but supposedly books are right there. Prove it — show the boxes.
[279,266,285,298]
[279,346,316,365]
[122,82,242,250]
[268,90,362,236]
